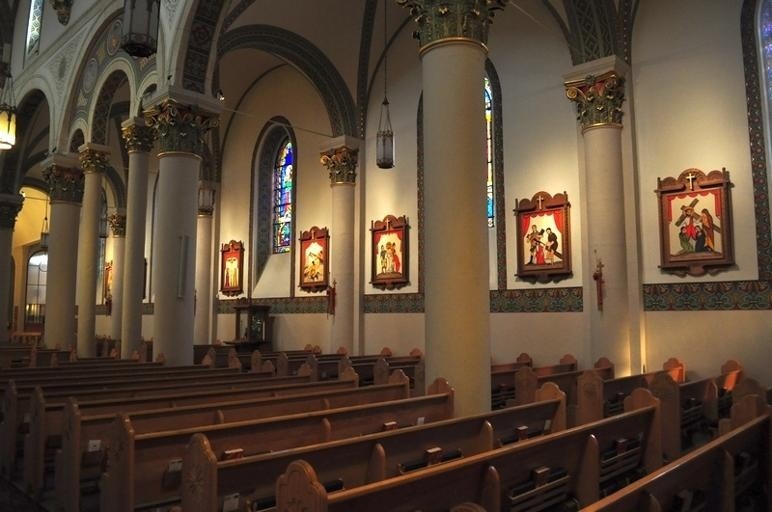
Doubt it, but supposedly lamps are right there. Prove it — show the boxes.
[590,247,606,313]
[374,8,400,170]
[0,15,16,151]
[29,193,50,266]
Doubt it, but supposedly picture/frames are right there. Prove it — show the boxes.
[511,191,573,285]
[218,238,246,297]
[367,213,412,292]
[650,167,740,278]
[297,226,330,293]
[100,256,147,304]
